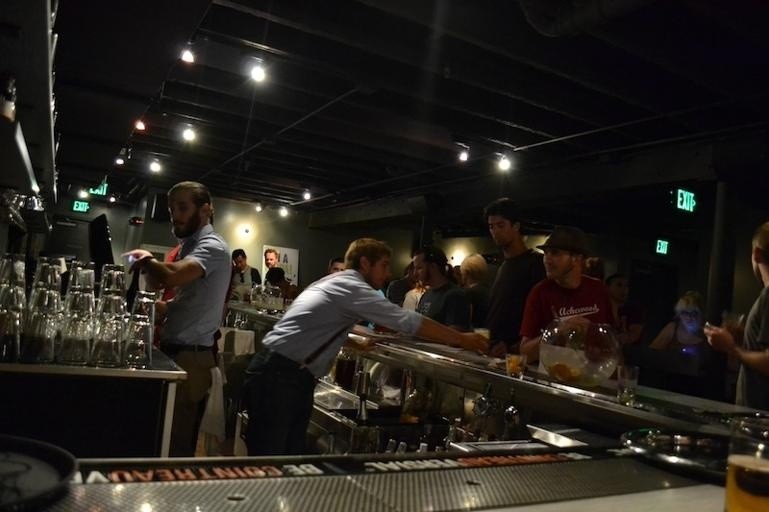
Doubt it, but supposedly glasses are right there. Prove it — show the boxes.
[239,273,243,282]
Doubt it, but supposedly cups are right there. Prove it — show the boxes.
[505,352,528,380]
[616,363,640,408]
[723,415,769,511]
[720,308,745,328]
[1,254,157,368]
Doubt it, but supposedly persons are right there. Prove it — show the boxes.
[266,266,303,304]
[231,247,261,300]
[264,249,278,268]
[328,257,345,273]
[645,291,722,401]
[386,197,646,370]
[122,180,231,458]
[244,236,489,456]
[703,222,768,456]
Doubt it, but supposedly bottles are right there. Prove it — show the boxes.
[382,439,443,454]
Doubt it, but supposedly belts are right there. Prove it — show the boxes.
[161,343,208,354]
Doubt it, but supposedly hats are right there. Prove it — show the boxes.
[535,225,592,255]
